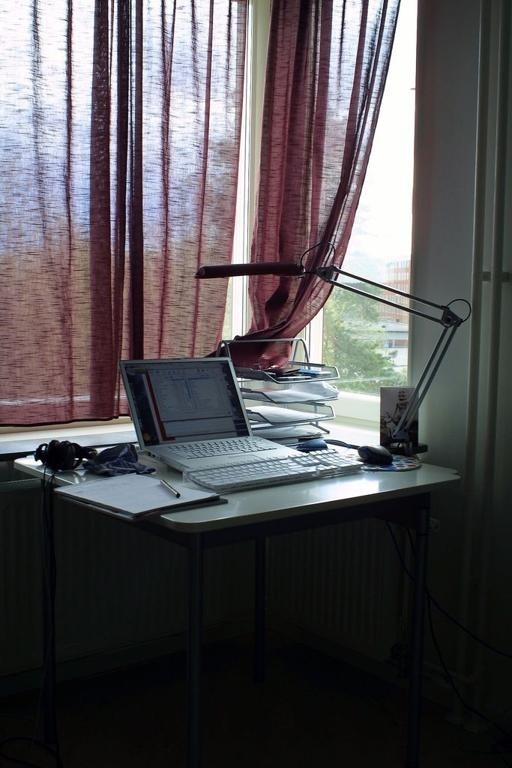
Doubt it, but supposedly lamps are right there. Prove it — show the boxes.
[196,261,471,456]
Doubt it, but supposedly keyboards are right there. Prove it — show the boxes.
[182,448,366,495]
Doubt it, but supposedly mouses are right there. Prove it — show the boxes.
[358,445,393,465]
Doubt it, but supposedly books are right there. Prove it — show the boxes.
[53,492,229,524]
[53,471,222,518]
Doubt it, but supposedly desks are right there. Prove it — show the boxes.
[14,439,462,753]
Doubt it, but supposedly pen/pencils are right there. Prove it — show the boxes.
[160,479,181,498]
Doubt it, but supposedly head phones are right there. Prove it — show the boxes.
[34,440,97,472]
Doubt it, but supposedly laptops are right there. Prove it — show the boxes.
[119,357,305,472]
